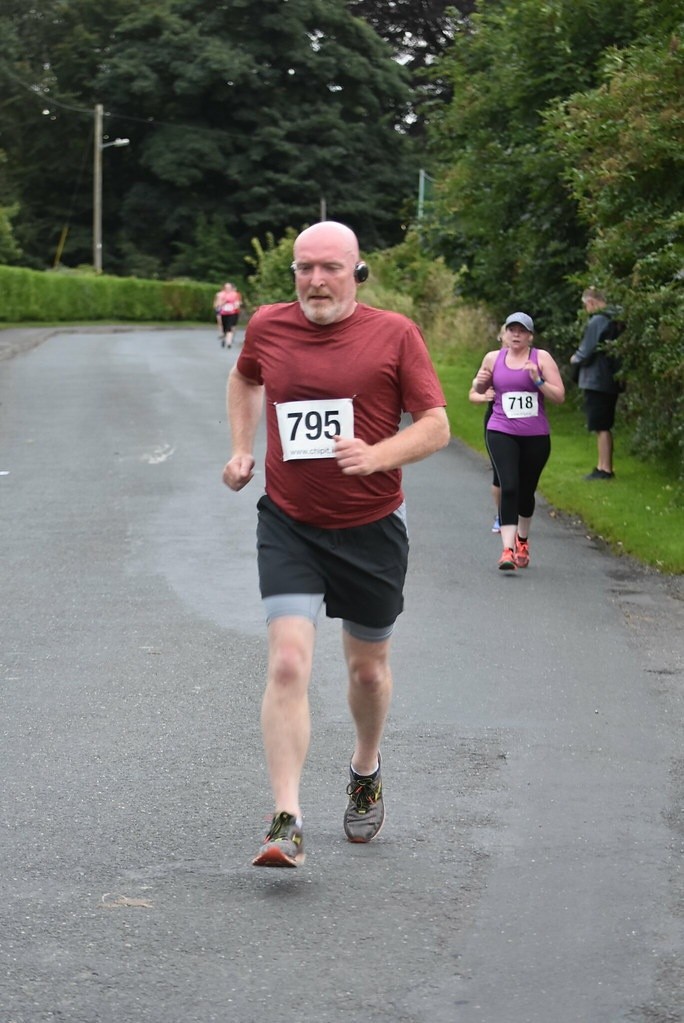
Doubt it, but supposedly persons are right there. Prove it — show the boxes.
[223,224,451,867]
[213,282,241,349]
[468,311,566,571]
[569,287,625,480]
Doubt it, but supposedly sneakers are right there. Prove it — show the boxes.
[252,810,305,867]
[498,548,518,571]
[514,531,530,568]
[343,747,386,843]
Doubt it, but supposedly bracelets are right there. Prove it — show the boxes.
[534,378,545,386]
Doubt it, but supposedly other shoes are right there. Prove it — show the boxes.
[585,467,615,480]
[491,514,500,533]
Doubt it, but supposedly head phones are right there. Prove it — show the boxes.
[290,262,369,282]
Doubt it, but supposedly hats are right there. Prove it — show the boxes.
[505,312,533,334]
